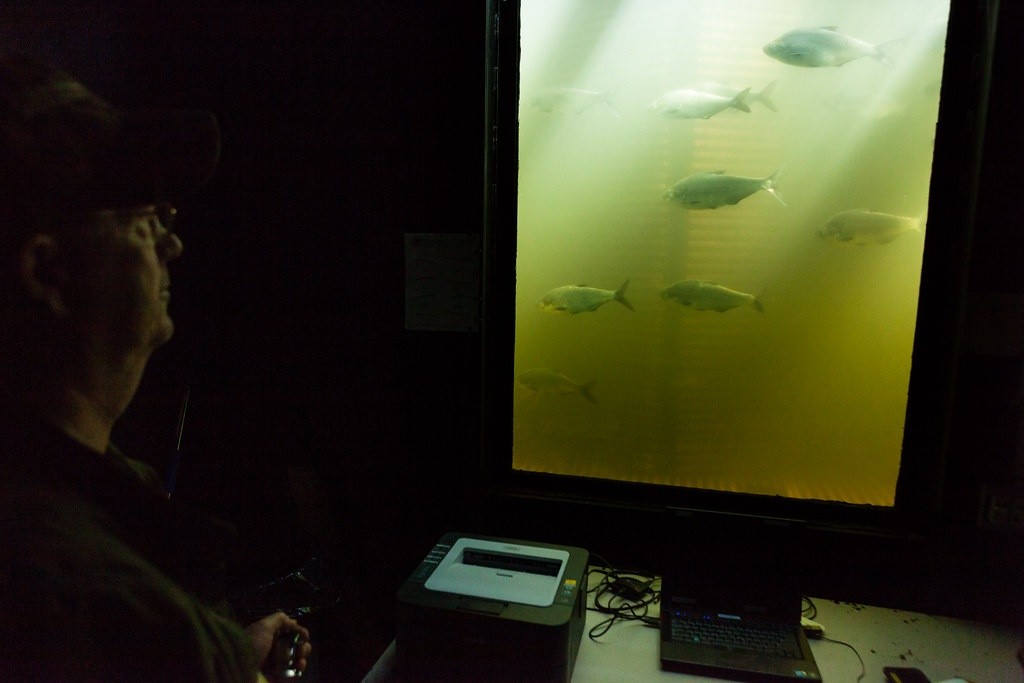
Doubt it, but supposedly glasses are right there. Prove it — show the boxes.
[78,204,182,237]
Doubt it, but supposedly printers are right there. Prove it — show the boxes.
[396,529,587,683]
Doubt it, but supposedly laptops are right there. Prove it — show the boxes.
[657,505,824,683]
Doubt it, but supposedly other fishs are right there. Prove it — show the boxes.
[656,279,770,316]
[537,279,637,316]
[515,369,601,406]
[762,25,901,70]
[654,155,793,210]
[655,74,782,122]
[813,206,928,247]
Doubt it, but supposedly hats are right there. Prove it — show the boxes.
[2,71,222,233]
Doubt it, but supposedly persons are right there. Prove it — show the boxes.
[0,78,310,683]
